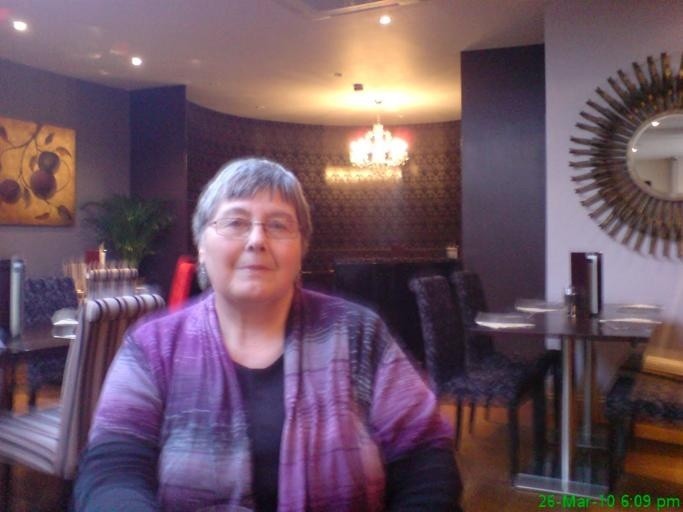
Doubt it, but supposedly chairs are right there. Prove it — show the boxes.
[302,257,683,492]
[0,256,196,512]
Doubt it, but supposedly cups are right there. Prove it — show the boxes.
[0,258,25,338]
[565,287,578,316]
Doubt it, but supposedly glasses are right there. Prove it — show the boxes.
[207,218,304,238]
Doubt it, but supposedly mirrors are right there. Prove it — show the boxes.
[569,52,683,256]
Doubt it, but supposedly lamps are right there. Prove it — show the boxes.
[349,100,409,168]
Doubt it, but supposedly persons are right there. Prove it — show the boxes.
[73,157,462,512]
